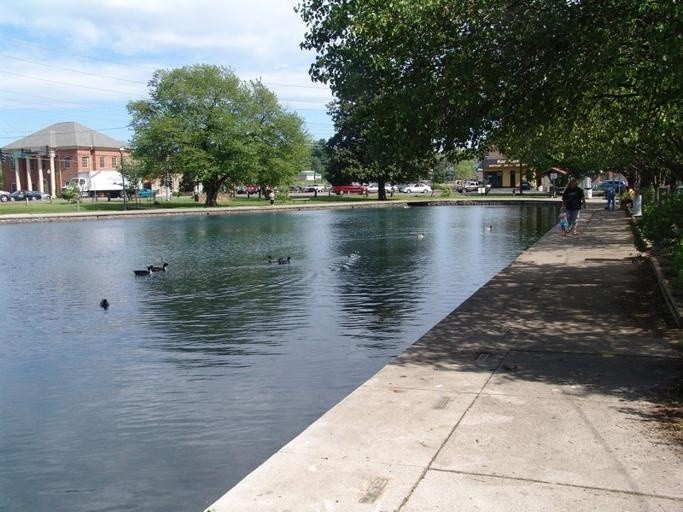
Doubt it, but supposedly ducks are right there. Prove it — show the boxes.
[148,263,168,274]
[268,256,279,263]
[348,250,357,258]
[485,225,493,231]
[278,256,292,265]
[100,299,109,309]
[416,233,425,241]
[340,261,354,272]
[133,265,154,279]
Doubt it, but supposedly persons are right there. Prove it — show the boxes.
[603,180,616,216]
[561,177,587,236]
[623,186,634,212]
[267,190,275,207]
[557,206,570,241]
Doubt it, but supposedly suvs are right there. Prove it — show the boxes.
[598,180,626,192]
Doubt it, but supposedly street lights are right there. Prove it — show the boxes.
[118,147,126,210]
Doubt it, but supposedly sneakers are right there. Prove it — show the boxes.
[561,228,578,240]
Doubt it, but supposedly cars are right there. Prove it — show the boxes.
[0,189,50,202]
[456,181,491,193]
[234,182,432,197]
[137,188,155,198]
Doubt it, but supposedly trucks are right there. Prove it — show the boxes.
[61,170,143,198]
